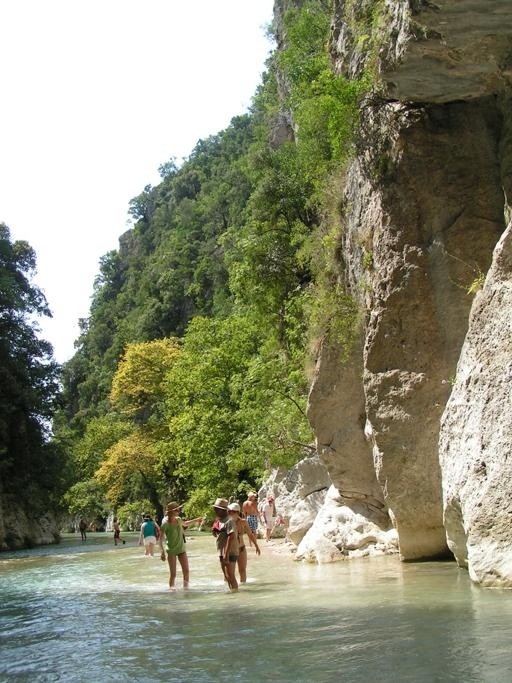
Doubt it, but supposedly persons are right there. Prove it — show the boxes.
[79,518,88,541]
[139,490,278,590]
[112,516,126,546]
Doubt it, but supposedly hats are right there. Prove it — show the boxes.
[165,501,183,516]
[144,514,151,520]
[227,502,241,512]
[210,497,231,512]
